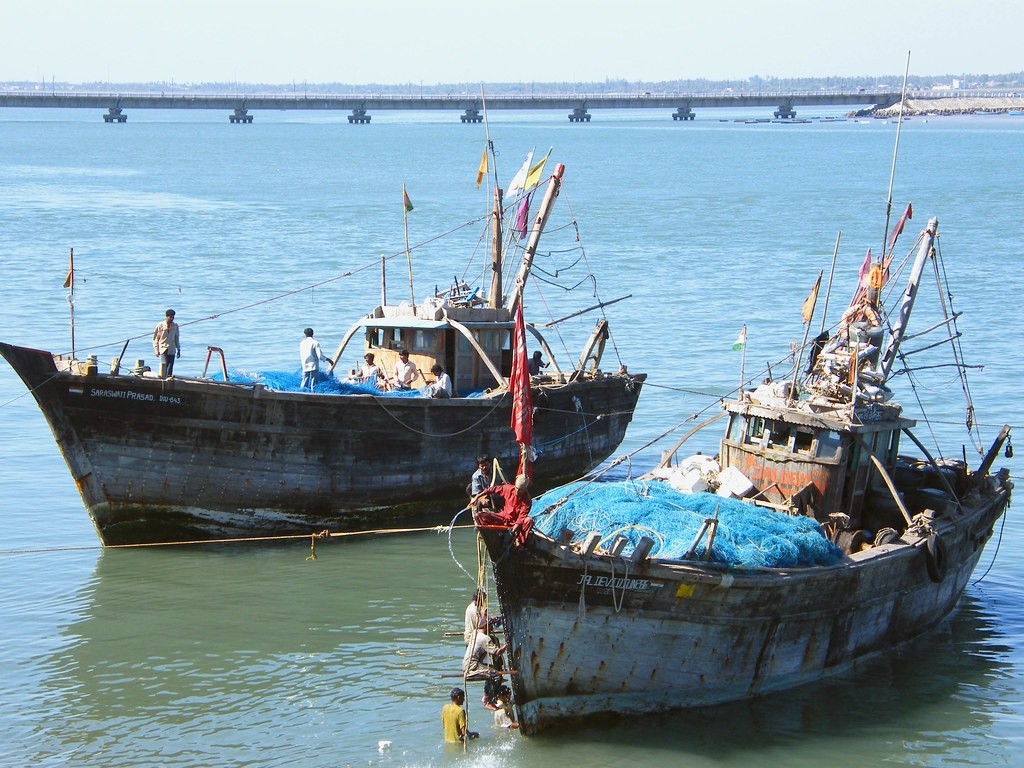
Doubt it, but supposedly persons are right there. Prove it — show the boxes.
[393,350,419,392]
[462,589,521,730]
[441,688,479,744]
[427,365,452,399]
[471,454,533,555]
[527,351,554,376]
[153,309,180,377]
[349,353,388,396]
[299,328,334,392]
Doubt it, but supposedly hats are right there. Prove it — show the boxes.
[515,475,529,489]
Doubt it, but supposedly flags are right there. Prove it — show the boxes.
[510,299,534,444]
[857,248,891,297]
[802,275,820,323]
[888,202,914,248]
[477,146,547,237]
[404,190,414,212]
[732,325,748,350]
[63,270,75,287]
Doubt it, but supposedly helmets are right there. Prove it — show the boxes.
[478,617,492,628]
[472,589,487,599]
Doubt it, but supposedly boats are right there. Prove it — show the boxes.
[975,110,1001,115]
[1009,112,1024,115]
[0,84,648,548]
[478,52,1016,740]
